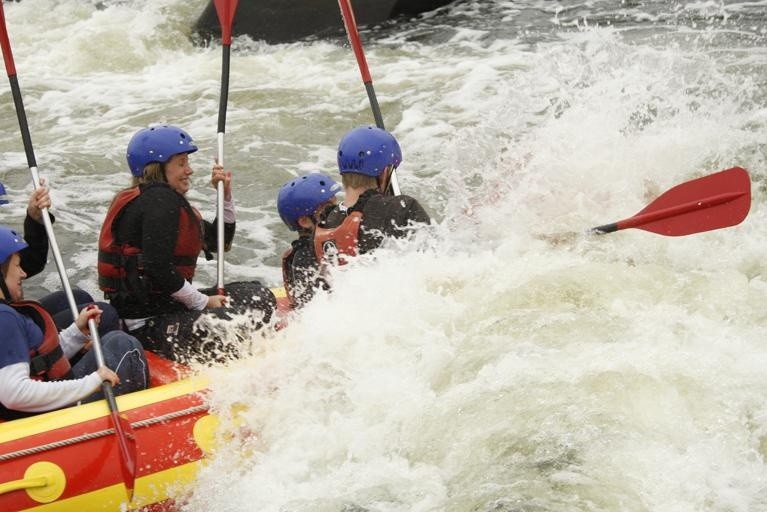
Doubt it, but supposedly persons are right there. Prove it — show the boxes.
[276,170,345,307]
[0,179,55,279]
[95,125,275,368]
[302,124,430,287]
[0,227,148,420]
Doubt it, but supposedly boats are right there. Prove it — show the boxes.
[0,287,289,512]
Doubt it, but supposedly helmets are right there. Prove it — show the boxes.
[336,124,402,177]
[276,172,343,232]
[0,225,30,266]
[127,124,199,177]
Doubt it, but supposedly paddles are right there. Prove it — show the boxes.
[0,20,136,489]
[211,0,239,305]
[541,166,750,237]
[338,0,401,197]
